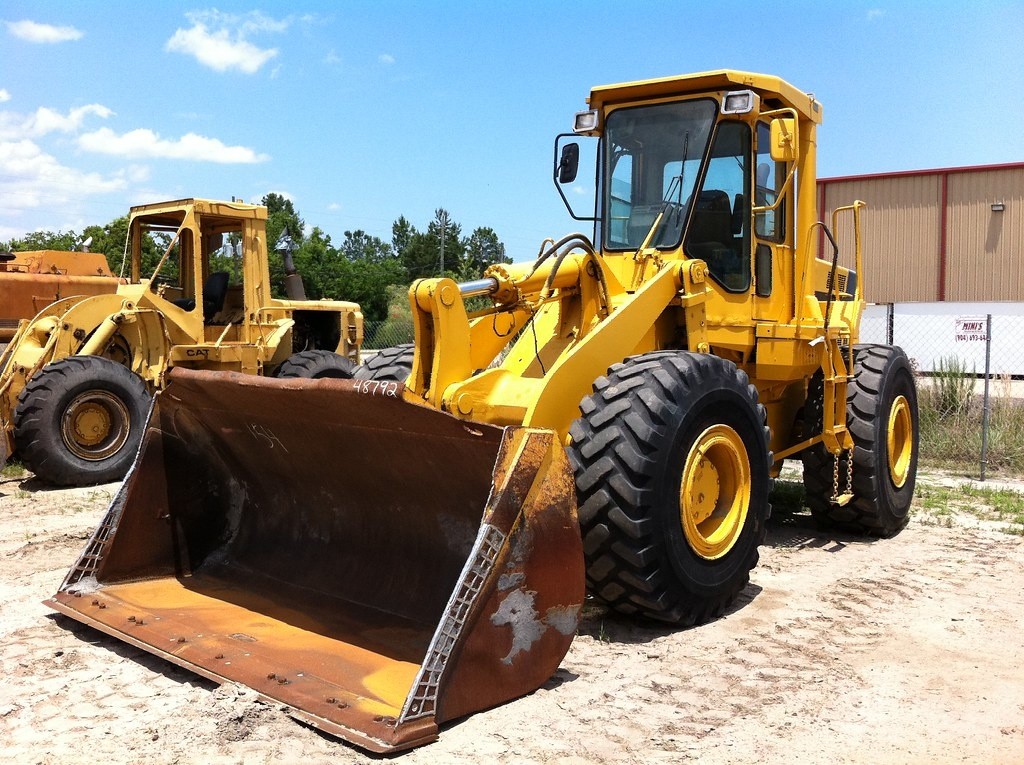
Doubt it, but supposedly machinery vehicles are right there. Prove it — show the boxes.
[0,235,130,371]
[38,69,921,754]
[0,196,363,487]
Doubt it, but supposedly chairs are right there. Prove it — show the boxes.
[168,271,231,326]
[656,190,736,277]
